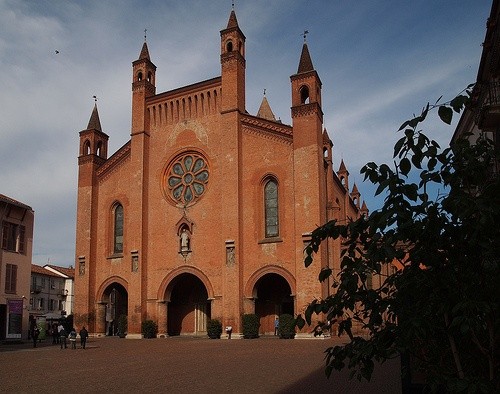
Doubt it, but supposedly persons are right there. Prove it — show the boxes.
[226,247,236,267]
[274,318,281,337]
[68,328,77,351]
[180,228,188,252]
[79,325,89,350]
[32,325,40,349]
[51,322,68,349]
